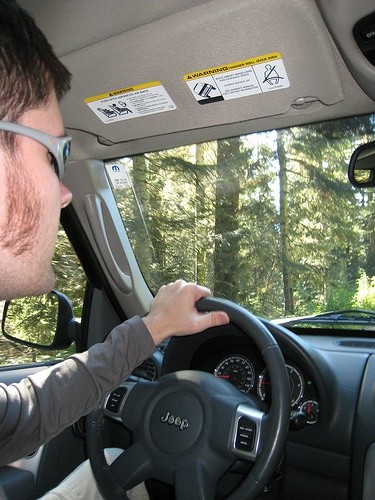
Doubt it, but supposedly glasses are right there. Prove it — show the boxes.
[0,120,72,178]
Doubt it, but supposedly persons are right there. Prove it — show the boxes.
[1,0,230,500]
